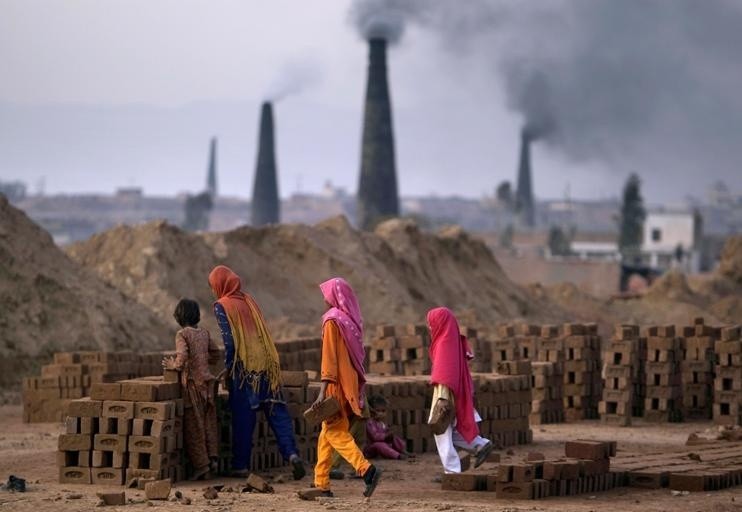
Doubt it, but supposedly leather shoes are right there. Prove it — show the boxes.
[187,460,248,480]
[292,458,305,479]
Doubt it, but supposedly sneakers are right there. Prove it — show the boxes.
[474,445,495,468]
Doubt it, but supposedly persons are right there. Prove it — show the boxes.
[364,397,416,459]
[425,308,492,481]
[207,266,306,481]
[161,299,226,480]
[297,277,381,501]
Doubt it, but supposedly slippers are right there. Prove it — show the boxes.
[363,472,383,496]
